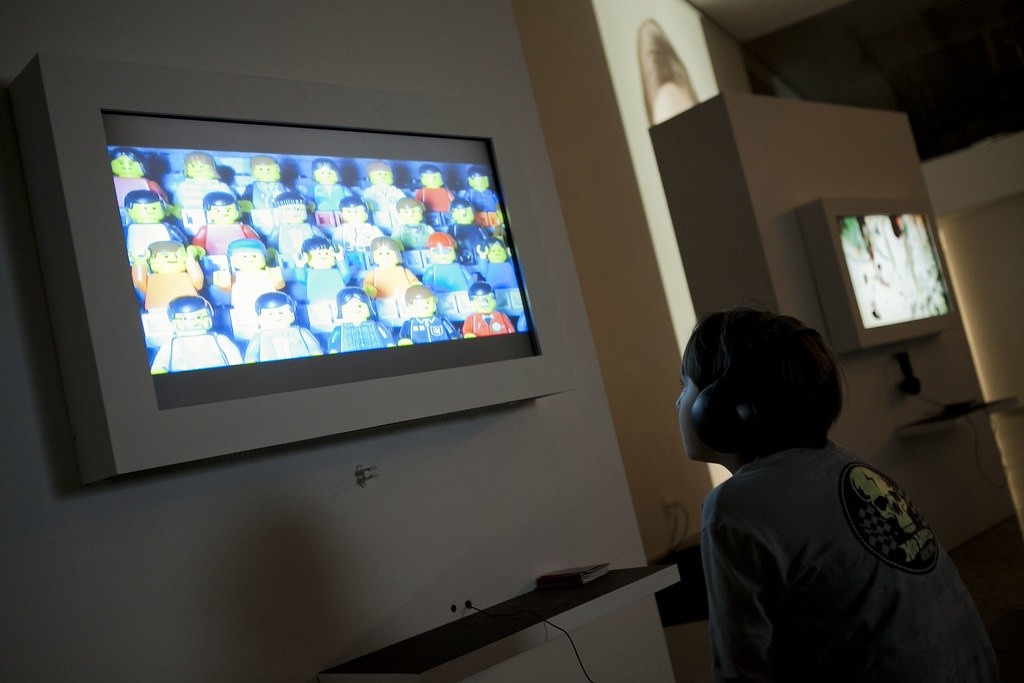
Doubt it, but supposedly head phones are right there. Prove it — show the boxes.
[894,354,921,395]
[690,308,760,452]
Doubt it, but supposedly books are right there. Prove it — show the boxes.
[536,563,610,586]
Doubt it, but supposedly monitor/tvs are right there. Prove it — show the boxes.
[795,196,955,355]
[10,54,578,485]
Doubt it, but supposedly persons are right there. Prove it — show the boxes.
[675,308,999,683]
[111,144,526,377]
